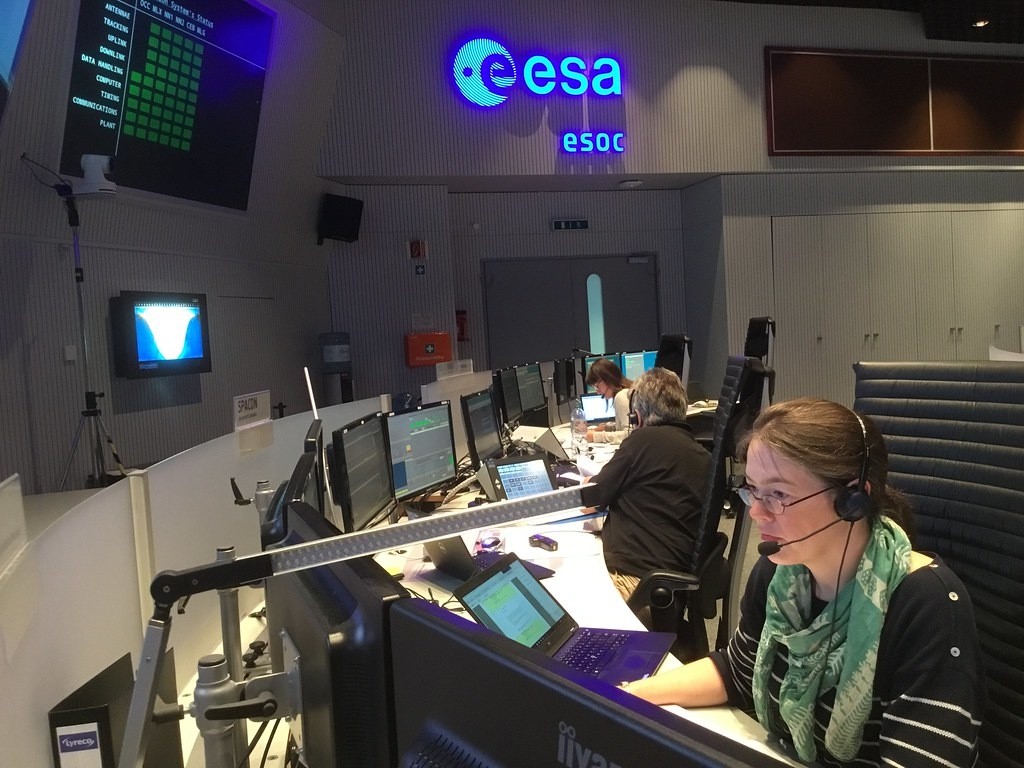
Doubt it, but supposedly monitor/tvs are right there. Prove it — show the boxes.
[120,289,212,377]
[261,348,794,768]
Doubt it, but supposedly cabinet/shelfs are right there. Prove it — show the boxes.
[772,210,1024,411]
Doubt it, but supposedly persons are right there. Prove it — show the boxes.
[575,357,636,445]
[579,366,725,632]
[615,395,985,768]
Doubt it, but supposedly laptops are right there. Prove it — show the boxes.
[422,535,555,582]
[451,551,676,688]
[580,393,616,426]
[484,453,560,502]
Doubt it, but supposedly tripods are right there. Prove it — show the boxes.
[52,182,128,493]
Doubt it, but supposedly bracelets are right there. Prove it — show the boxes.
[621,680,631,695]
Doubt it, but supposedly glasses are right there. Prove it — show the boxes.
[736,477,857,515]
[592,379,603,391]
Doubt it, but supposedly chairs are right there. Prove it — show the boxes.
[625,318,1024,768]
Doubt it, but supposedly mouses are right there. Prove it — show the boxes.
[480,537,500,548]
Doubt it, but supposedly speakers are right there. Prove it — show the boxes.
[316,194,365,244]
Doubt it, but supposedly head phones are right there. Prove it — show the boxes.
[628,388,643,429]
[835,407,871,522]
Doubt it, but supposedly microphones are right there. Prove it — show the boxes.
[601,388,609,399]
[757,506,861,556]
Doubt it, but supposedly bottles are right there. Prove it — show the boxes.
[570,399,588,460]
[319,332,351,372]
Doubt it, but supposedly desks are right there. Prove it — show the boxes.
[0,335,823,768]
